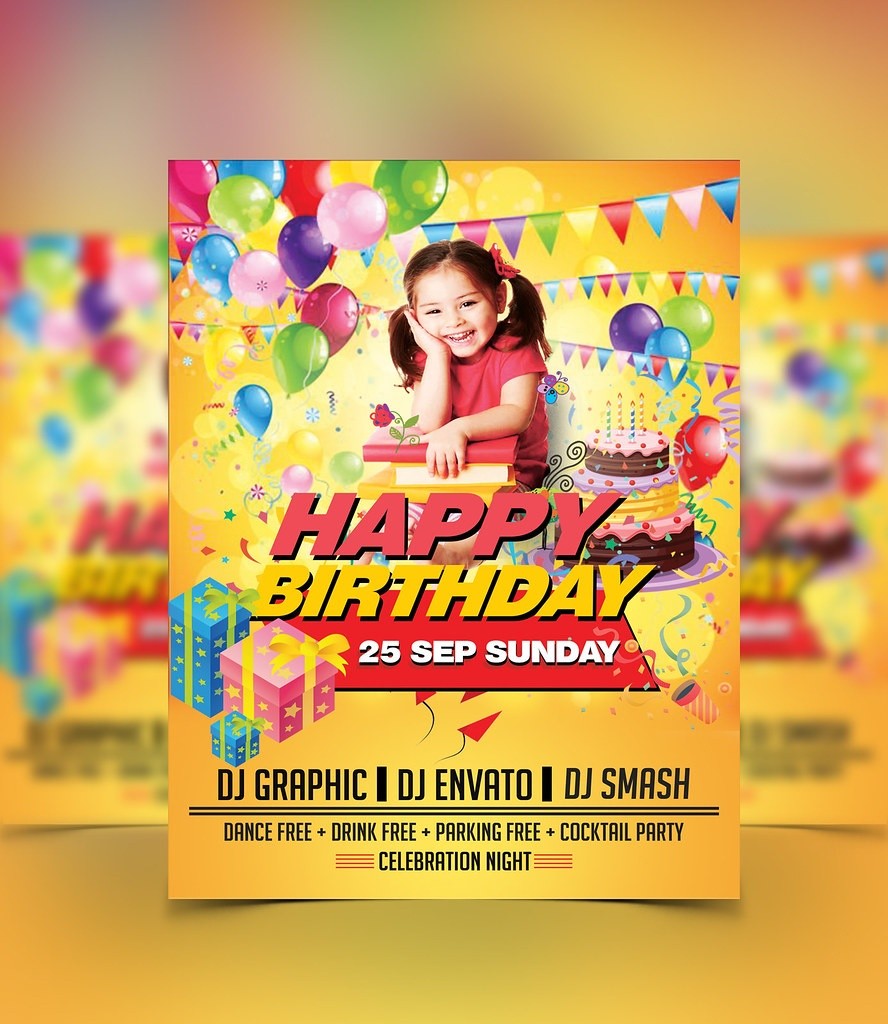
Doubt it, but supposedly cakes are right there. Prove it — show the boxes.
[554,428,695,575]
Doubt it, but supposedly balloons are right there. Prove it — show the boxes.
[0,159,878,493]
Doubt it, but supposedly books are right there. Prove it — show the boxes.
[358,423,526,511]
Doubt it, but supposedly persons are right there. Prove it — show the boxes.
[386,237,554,493]
